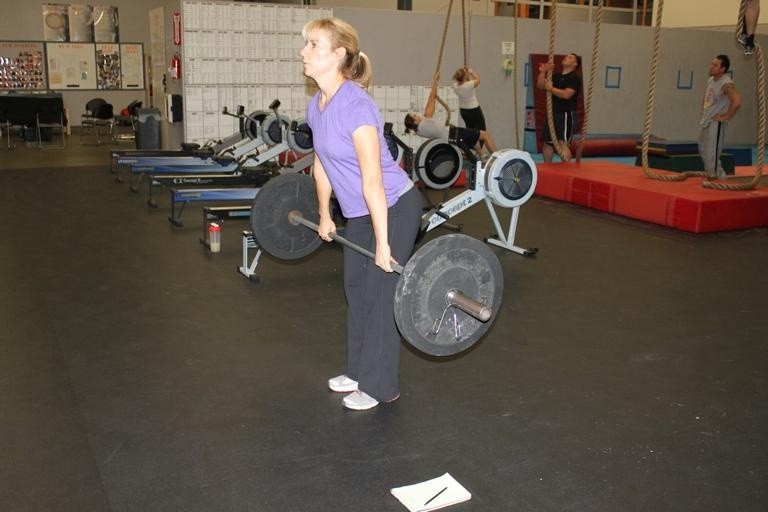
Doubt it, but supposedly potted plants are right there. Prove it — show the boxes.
[523,62,528,87]
[603,64,621,89]
[675,67,695,90]
[727,70,733,81]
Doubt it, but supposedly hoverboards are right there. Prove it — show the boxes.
[250,173,504,356]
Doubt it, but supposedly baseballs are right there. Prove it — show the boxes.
[170,53,180,80]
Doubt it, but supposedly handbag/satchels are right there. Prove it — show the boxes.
[467,68,473,73]
[551,87,553,92]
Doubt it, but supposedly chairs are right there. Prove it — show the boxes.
[329,375,359,391]
[341,387,401,410]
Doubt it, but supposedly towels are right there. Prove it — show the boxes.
[136,106,161,151]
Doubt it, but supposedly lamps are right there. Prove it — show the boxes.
[0,90,145,151]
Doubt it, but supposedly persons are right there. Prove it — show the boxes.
[448,65,485,155]
[299,17,422,411]
[401,72,498,162]
[738,1,760,56]
[697,55,741,180]
[537,53,583,163]
